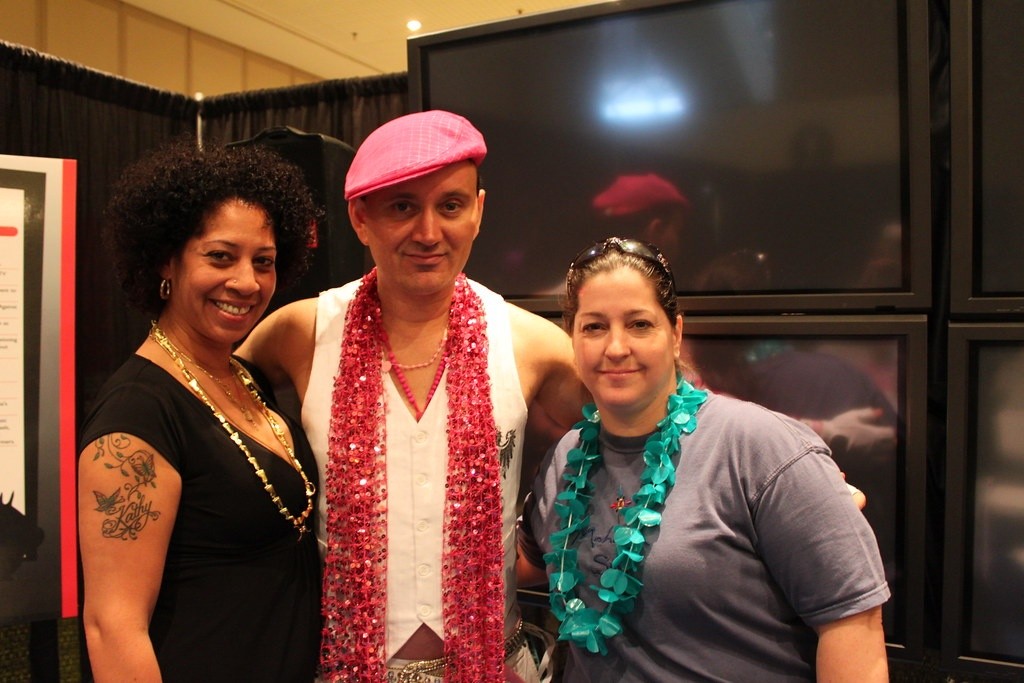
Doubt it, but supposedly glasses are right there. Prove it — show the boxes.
[572,237,670,283]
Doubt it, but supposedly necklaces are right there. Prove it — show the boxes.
[148,323,314,539]
[544,379,707,654]
[318,263,511,682]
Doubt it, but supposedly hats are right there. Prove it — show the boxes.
[344,110,487,201]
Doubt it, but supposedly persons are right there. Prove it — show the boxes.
[513,237,891,683]
[533,170,902,483]
[232,108,867,683]
[74,147,324,683]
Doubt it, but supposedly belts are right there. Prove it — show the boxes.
[384,606,525,682]
